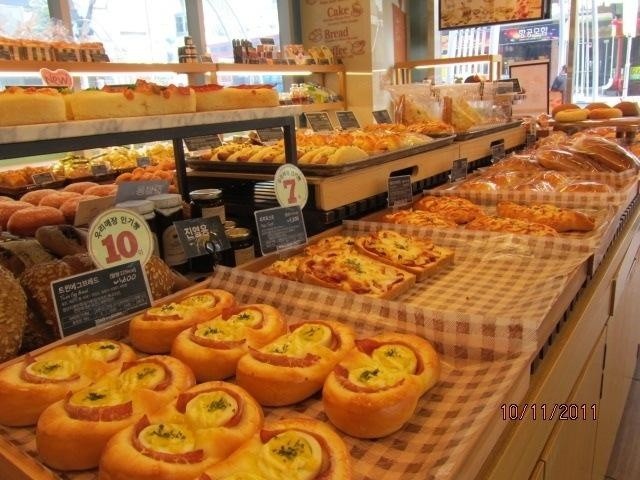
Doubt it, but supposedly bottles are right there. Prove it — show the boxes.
[280,83,329,104]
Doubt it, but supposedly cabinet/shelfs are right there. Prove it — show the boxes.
[0,180,616,480]
[586,209,640,480]
[0,52,353,121]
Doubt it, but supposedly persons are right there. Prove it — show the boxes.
[552,65,567,104]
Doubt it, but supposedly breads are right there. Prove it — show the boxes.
[0,284,442,480]
[196,122,433,169]
[360,81,556,144]
[384,193,596,239]
[269,222,455,303]
[0,83,280,124]
[453,122,639,197]
[551,101,640,122]
[0,144,188,361]
